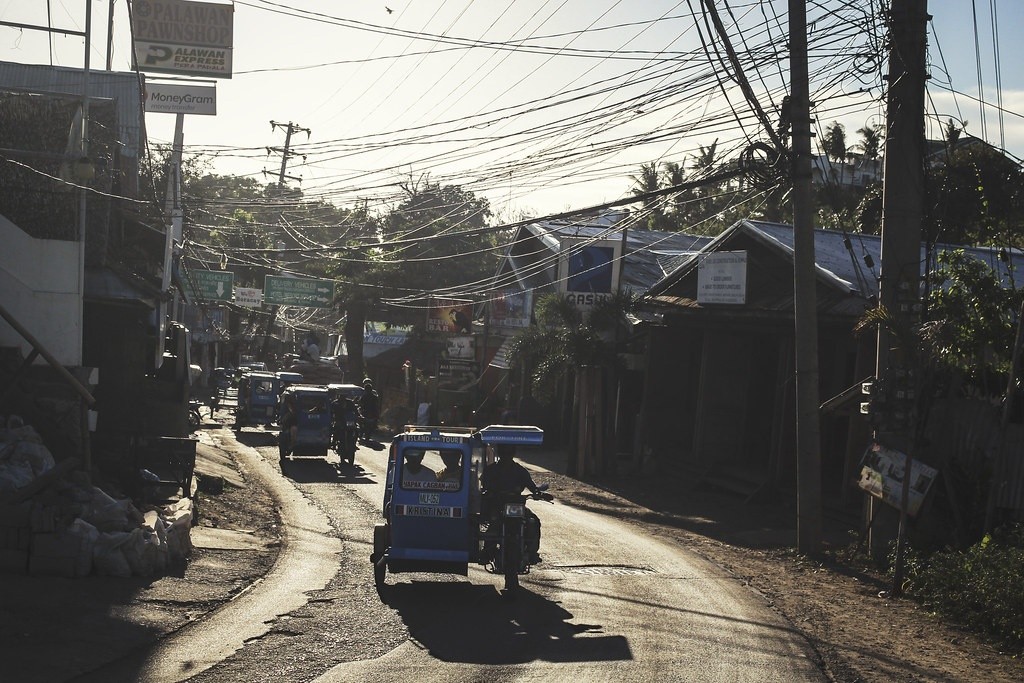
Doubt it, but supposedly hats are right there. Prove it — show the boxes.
[404,448,426,456]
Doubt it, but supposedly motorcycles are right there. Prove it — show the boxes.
[231,361,303,435]
[369,423,554,599]
[275,383,367,466]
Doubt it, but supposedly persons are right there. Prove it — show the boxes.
[389,447,476,502]
[448,309,470,333]
[280,399,328,424]
[351,378,382,430]
[299,333,322,363]
[330,394,363,451]
[481,443,553,564]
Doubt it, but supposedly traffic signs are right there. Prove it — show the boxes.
[264,275,334,309]
[182,267,234,302]
